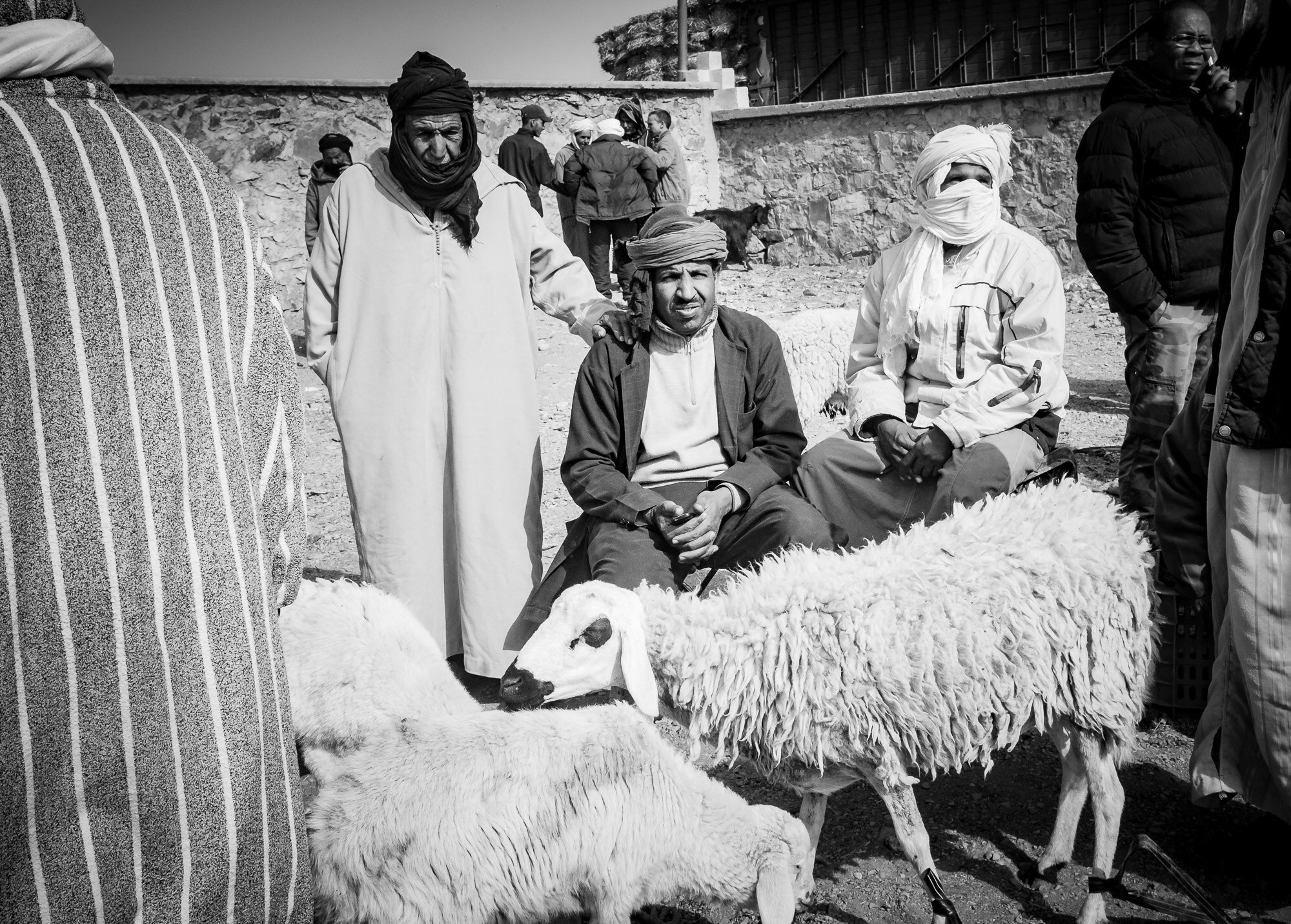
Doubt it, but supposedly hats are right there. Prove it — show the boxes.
[596,118,625,138]
[911,122,1015,247]
[387,49,483,249]
[625,204,730,330]
[317,133,353,155]
[616,96,645,141]
[522,105,553,122]
[569,117,594,151]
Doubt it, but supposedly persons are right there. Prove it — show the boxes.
[1075,2,1291,822]
[788,121,1070,554]
[306,49,645,680]
[0,0,314,924]
[497,97,691,307]
[559,204,834,599]
[306,133,355,259]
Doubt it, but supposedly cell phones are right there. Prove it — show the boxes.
[672,512,696,525]
[1209,55,1220,93]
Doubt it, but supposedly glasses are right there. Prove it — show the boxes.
[1165,34,1218,50]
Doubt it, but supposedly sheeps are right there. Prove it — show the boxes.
[304,701,816,924]
[274,574,484,790]
[498,478,1159,924]
[692,200,769,272]
